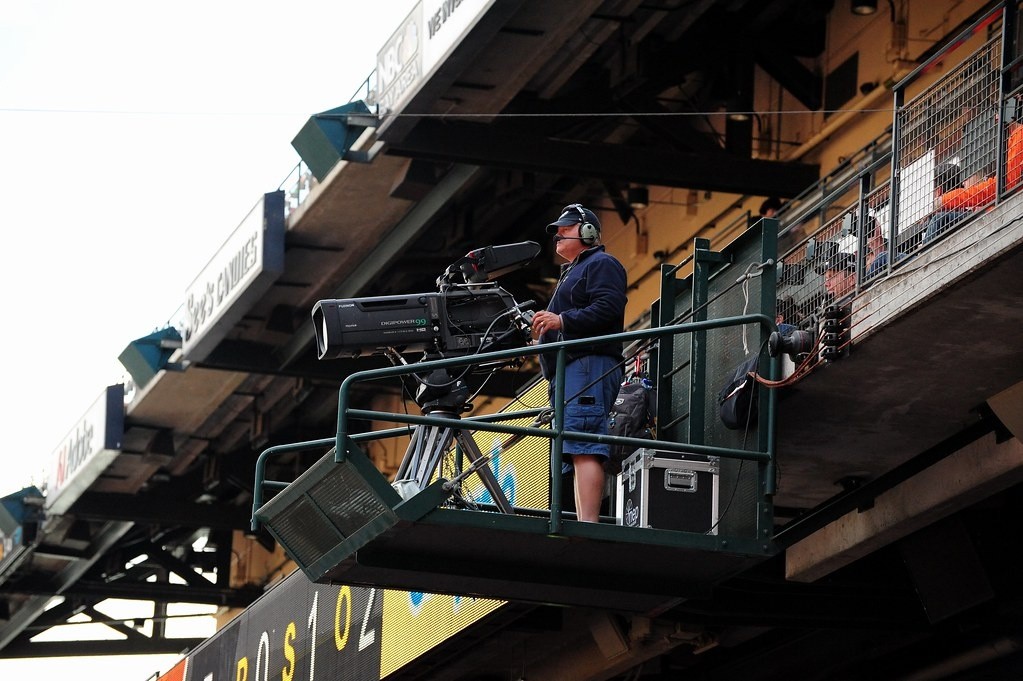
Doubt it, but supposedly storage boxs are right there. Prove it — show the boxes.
[620,449,720,535]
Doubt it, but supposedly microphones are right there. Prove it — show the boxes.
[553,235,592,242]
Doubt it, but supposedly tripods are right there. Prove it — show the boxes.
[394,400,514,517]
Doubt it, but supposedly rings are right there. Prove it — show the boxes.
[541,322,544,327]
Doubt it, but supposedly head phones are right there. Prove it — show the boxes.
[561,203,598,245]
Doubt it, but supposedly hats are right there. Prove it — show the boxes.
[546,208,602,234]
[814,252,855,275]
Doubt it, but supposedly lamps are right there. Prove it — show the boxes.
[629,187,648,257]
[292,99,384,184]
[119,327,190,390]
[0,486,46,540]
[730,96,772,153]
[850,0,903,61]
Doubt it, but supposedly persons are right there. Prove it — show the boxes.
[530,204,626,522]
[759,94,1023,326]
